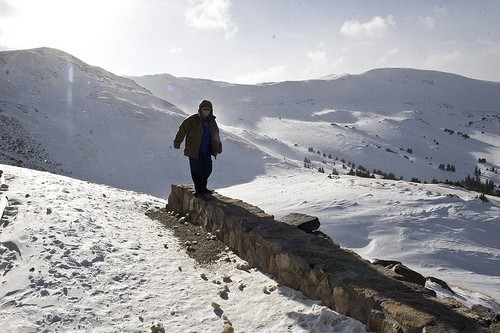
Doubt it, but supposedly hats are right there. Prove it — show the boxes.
[198,100,213,112]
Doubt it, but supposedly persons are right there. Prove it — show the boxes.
[174,99,223,201]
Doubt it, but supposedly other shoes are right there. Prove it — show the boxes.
[196,187,211,196]
